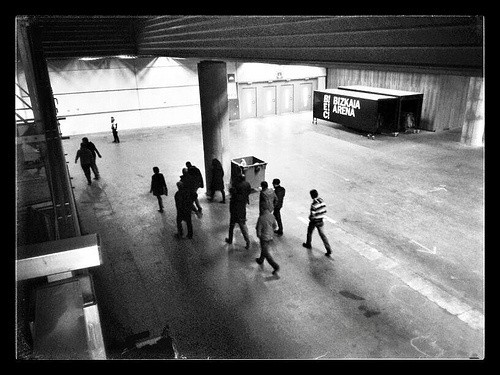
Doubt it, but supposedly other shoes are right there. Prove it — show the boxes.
[273,265,280,273]
[225,237,231,243]
[187,234,191,239]
[87,182,91,185]
[303,242,311,249]
[208,198,212,203]
[256,258,262,264]
[245,244,249,248]
[274,230,282,235]
[174,233,183,238]
[199,207,202,214]
[94,177,98,180]
[325,251,331,256]
[158,209,162,213]
[196,212,201,219]
[113,140,116,143]
[220,201,225,204]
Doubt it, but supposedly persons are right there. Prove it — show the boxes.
[172,162,204,240]
[225,174,262,250]
[111,117,120,143]
[207,159,225,203]
[303,190,332,256]
[255,208,280,274]
[258,181,279,232]
[150,167,167,213]
[75,138,101,186]
[272,179,285,236]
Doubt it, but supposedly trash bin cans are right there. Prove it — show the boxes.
[231,156,267,190]
